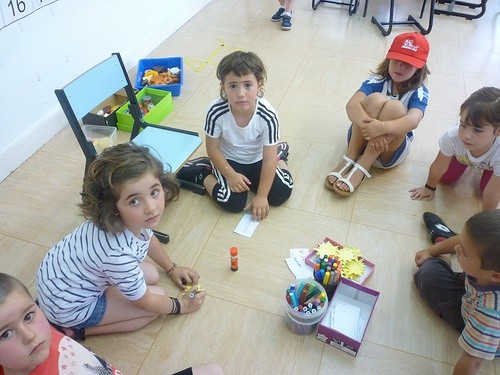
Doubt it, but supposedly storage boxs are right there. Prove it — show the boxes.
[82,86,139,136]
[306,236,376,286]
[134,56,184,96]
[314,277,380,357]
[115,87,173,134]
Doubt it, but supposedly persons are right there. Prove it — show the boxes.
[0,272,224,375]
[409,86,500,211]
[413,208,500,375]
[325,32,429,196]
[175,51,294,221]
[35,141,206,339]
[271,0,295,30]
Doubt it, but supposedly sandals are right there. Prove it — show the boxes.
[325,155,356,187]
[333,163,371,196]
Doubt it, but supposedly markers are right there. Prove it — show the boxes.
[283,278,328,334]
[189,290,197,299]
[313,253,343,284]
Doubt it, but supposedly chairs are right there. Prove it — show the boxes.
[55,53,206,243]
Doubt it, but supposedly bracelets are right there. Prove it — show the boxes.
[167,263,177,275]
[166,297,181,315]
[425,184,436,192]
[221,164,230,174]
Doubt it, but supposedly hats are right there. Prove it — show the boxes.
[386,31,429,68]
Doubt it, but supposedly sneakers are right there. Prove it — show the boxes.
[276,142,290,162]
[272,7,285,21]
[176,157,212,184]
[280,10,293,29]
[423,212,457,254]
